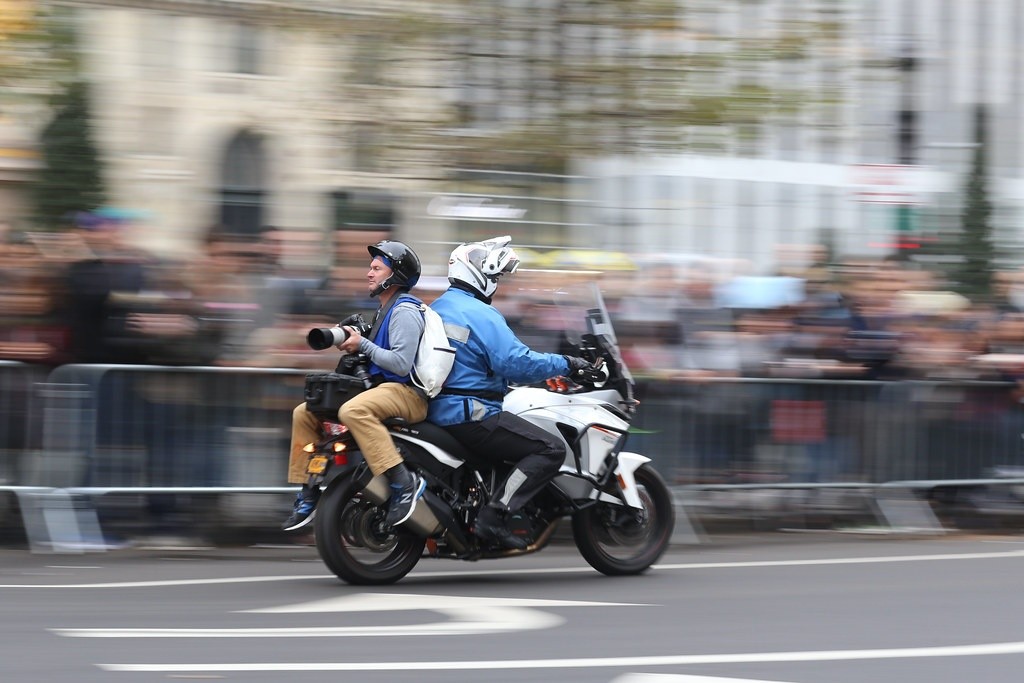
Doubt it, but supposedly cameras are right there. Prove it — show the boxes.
[307,313,373,351]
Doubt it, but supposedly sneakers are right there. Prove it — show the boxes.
[280,484,322,531]
[387,473,431,526]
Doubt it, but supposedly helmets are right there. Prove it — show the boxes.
[449,235,520,297]
[367,240,421,285]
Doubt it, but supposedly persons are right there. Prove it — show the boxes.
[281,241,428,531]
[427,235,593,550]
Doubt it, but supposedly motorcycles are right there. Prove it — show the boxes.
[302,283,677,587]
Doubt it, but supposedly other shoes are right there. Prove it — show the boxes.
[478,503,533,532]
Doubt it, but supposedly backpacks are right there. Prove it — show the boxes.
[410,304,457,397]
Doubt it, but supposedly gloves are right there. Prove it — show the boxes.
[563,355,600,387]
